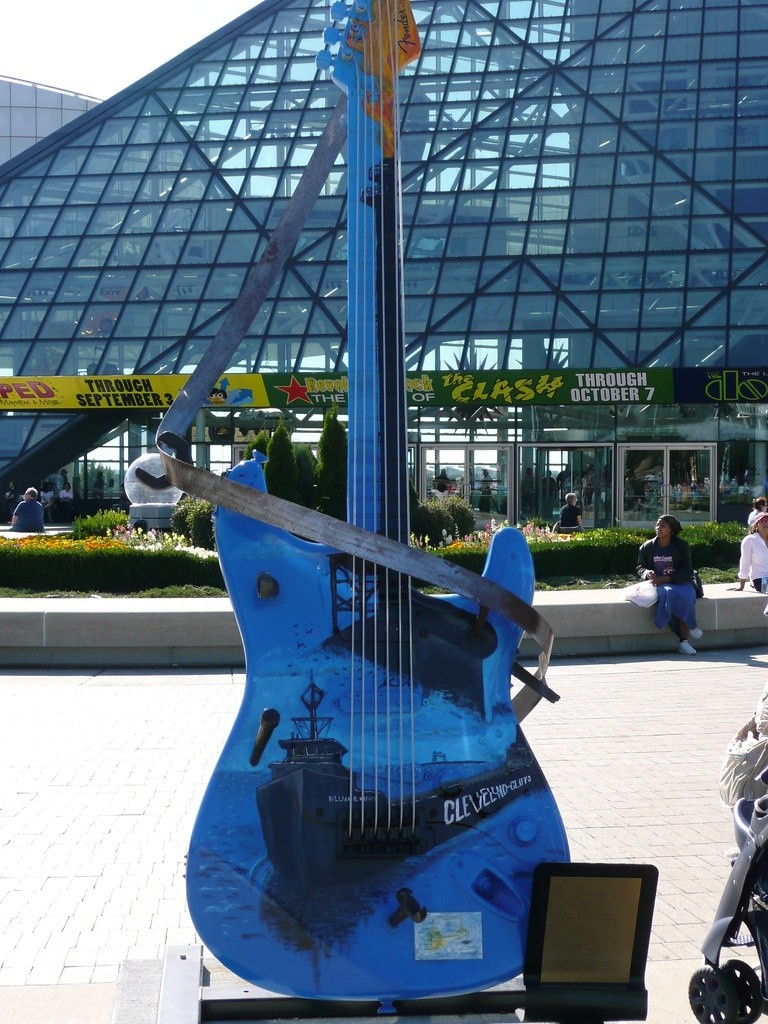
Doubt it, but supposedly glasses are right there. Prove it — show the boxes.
[762,505,768,508]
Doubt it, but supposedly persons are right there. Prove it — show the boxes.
[559,493,587,533]
[434,469,450,492]
[637,515,703,655]
[9,487,45,533]
[93,471,104,500]
[727,497,768,616]
[669,478,751,501]
[478,468,494,513]
[5,482,75,523]
[60,469,69,482]
[520,462,636,519]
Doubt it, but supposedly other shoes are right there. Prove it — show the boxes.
[49,518,53,523]
[764,604,768,617]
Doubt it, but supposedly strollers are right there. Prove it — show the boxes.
[686,764,768,1024]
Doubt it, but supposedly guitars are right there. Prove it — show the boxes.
[155,0,565,1004]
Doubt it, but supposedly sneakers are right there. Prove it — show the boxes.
[688,628,703,638]
[678,640,697,655]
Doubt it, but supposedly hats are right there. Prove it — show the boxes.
[754,511,768,525]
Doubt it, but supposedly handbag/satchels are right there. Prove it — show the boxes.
[623,577,658,608]
[719,716,768,806]
[691,570,704,599]
